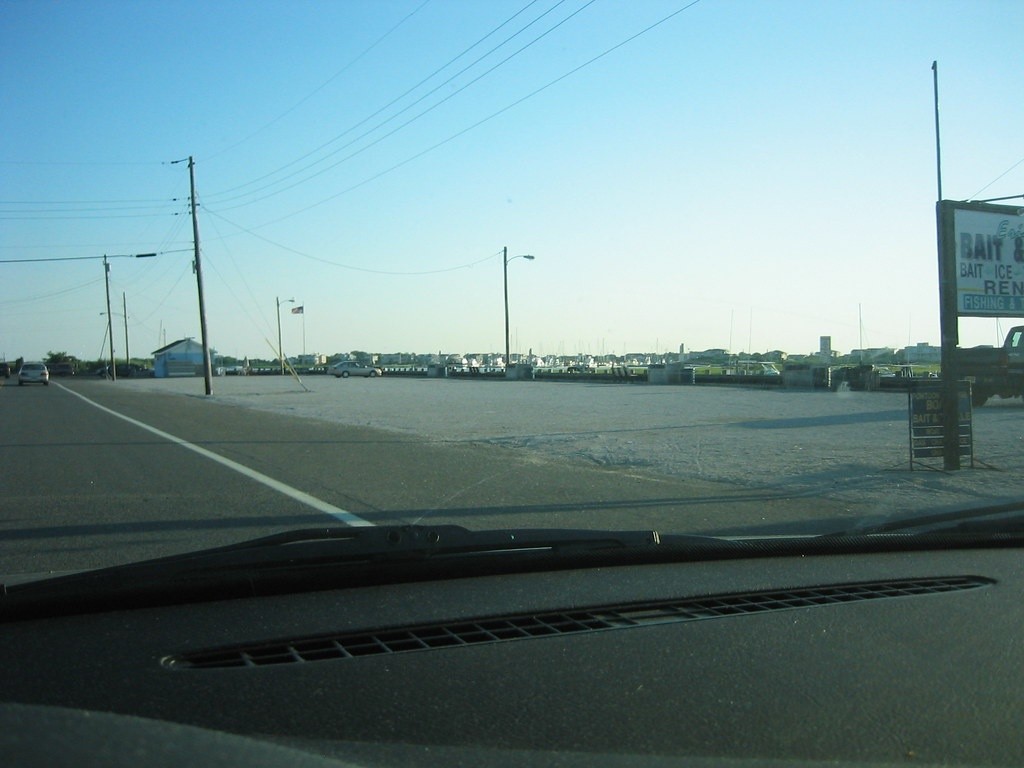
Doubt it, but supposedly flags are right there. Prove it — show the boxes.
[292,306,303,314]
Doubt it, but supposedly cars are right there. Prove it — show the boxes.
[326,361,382,378]
[876,367,896,377]
[19,362,50,385]
[47,364,75,376]
[0,362,11,377]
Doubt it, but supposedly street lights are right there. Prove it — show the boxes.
[276,297,295,375]
[100,312,130,366]
[503,246,535,363]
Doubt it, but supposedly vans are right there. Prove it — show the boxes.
[738,360,781,377]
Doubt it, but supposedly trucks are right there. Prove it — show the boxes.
[955,326,1024,411]
